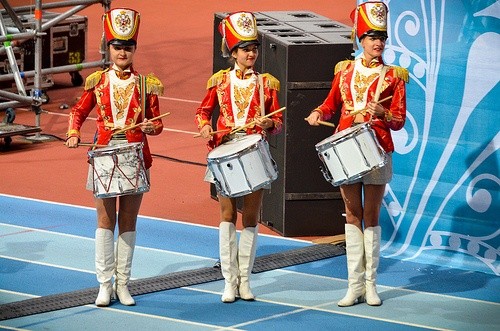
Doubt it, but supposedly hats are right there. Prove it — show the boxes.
[349,1,389,43]
[218,10,260,57]
[102,8,141,46]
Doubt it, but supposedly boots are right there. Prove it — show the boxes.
[337,224,366,306]
[95,228,114,306]
[238,225,258,300]
[112,231,136,305]
[364,226,381,305]
[218,222,238,303]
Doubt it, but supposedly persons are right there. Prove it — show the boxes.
[66,8,164,305]
[307,1,409,307]
[195,11,283,302]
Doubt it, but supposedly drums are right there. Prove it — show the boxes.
[315,120,388,187]
[86,141,150,199]
[206,133,280,198]
